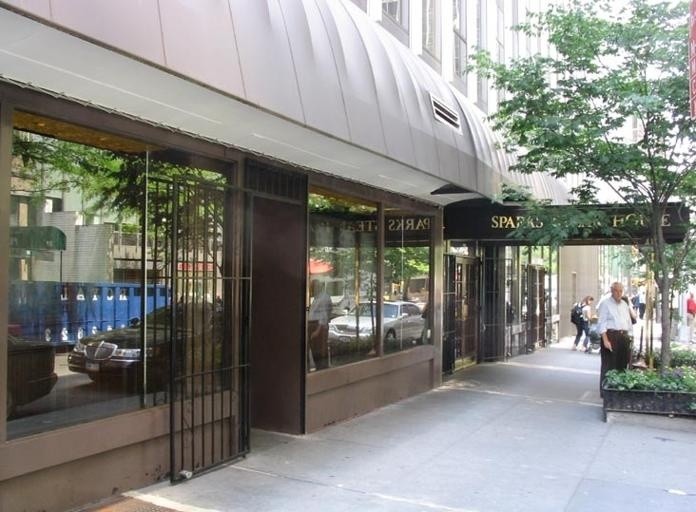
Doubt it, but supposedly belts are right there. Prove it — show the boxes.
[607,330,628,334]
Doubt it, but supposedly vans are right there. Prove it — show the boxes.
[310,276,377,312]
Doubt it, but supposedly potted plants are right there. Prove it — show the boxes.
[601,366,696,422]
[629,348,696,368]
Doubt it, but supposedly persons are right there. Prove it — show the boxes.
[621,294,637,348]
[58,283,128,302]
[592,281,638,397]
[306,277,333,370]
[566,295,594,354]
[685,292,696,345]
[630,292,639,315]
[581,314,602,353]
[637,291,648,319]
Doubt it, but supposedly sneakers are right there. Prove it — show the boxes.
[570,344,589,352]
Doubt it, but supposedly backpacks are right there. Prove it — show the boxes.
[570,302,586,323]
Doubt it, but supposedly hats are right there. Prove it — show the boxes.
[592,314,599,319]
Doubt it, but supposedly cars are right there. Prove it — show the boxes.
[68,303,226,390]
[327,300,426,350]
[6,331,58,418]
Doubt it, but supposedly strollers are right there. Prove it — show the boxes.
[588,316,601,353]
